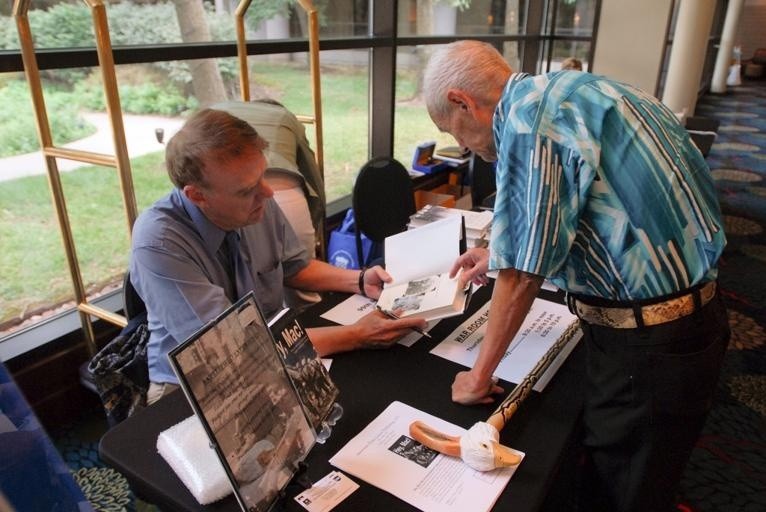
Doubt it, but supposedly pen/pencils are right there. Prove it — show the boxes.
[376,305,433,339]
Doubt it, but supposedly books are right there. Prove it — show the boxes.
[405,223,488,250]
[157,413,232,505]
[268,306,341,437]
[374,214,475,323]
[407,202,495,239]
[434,146,467,158]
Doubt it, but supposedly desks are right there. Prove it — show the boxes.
[98,280,596,511]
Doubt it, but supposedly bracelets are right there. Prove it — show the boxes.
[354,265,368,299]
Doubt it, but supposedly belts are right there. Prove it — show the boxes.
[563,277,718,331]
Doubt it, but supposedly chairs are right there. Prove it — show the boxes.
[121,270,147,320]
[469,151,498,211]
[353,153,416,268]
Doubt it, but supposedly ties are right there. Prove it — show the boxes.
[224,230,256,300]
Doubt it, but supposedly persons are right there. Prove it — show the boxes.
[419,35,733,512]
[287,356,335,421]
[125,107,428,407]
[199,97,328,317]
[561,57,583,72]
